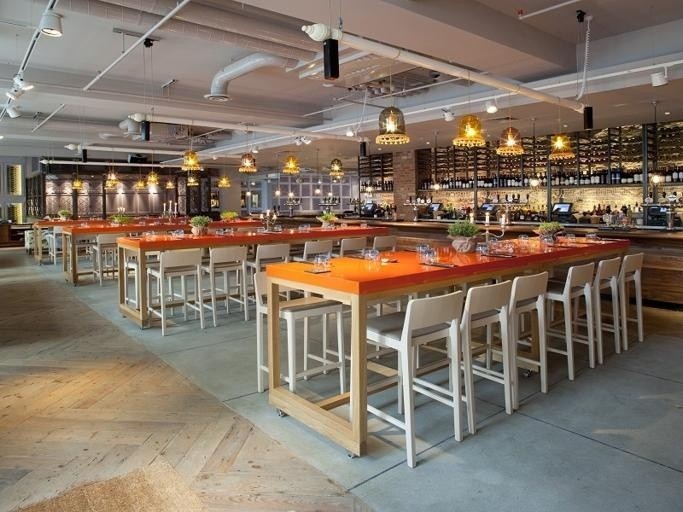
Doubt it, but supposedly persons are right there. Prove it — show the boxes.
[645,193,654,204]
[459,206,471,219]
[601,206,612,223]
[617,206,627,222]
[211,195,219,208]
[241,195,245,207]
[668,192,678,204]
[658,192,669,205]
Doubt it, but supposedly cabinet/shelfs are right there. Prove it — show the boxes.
[357,153,393,188]
[419,120,683,189]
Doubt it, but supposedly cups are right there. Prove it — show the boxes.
[312,228,596,273]
[145,222,311,242]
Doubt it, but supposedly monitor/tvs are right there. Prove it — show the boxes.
[649,206,675,217]
[479,203,499,214]
[551,203,573,214]
[364,203,377,213]
[428,202,443,213]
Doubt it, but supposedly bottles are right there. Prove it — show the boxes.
[591,201,640,216]
[421,165,683,191]
[359,176,396,192]
[526,203,548,212]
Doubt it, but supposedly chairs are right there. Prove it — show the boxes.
[24,230,50,255]
[46,223,398,339]
[254,233,644,467]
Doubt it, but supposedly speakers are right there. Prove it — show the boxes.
[141,121,150,141]
[323,39,339,79]
[583,106,593,130]
[82,149,88,162]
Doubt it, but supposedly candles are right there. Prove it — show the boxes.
[501,214,505,225]
[163,203,166,212]
[273,214,276,219]
[169,201,172,212]
[259,214,263,218]
[486,212,490,226]
[174,202,177,213]
[468,212,475,223]
[266,210,270,216]
[117,207,125,213]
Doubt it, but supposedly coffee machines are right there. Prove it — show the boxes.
[640,202,683,228]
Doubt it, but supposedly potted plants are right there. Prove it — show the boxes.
[58,210,72,221]
[316,212,339,228]
[187,215,213,235]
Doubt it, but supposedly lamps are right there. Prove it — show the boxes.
[39,10,63,38]
[140,42,152,142]
[453,80,485,147]
[12,74,34,91]
[300,135,312,144]
[294,137,302,146]
[485,99,499,115]
[239,130,257,173]
[323,2,340,80]
[251,145,259,153]
[345,126,355,137]
[576,9,594,130]
[444,112,455,122]
[496,90,524,156]
[359,95,366,157]
[182,126,201,170]
[548,105,575,160]
[72,165,199,188]
[329,139,345,176]
[376,60,410,145]
[283,135,300,173]
[5,103,23,120]
[650,66,669,87]
[218,168,231,187]
[6,88,20,101]
